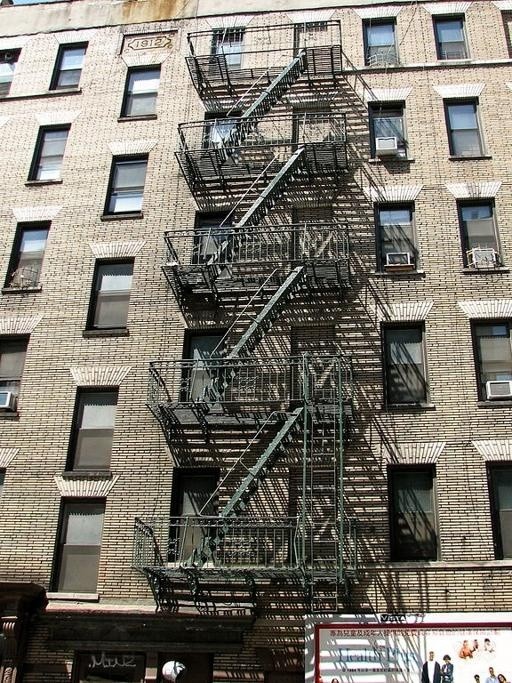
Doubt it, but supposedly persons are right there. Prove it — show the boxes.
[421,638,512,683]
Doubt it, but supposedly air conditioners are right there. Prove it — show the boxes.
[386,252,410,265]
[474,251,494,265]
[0,390,16,412]
[375,136,398,156]
[486,380,512,399]
